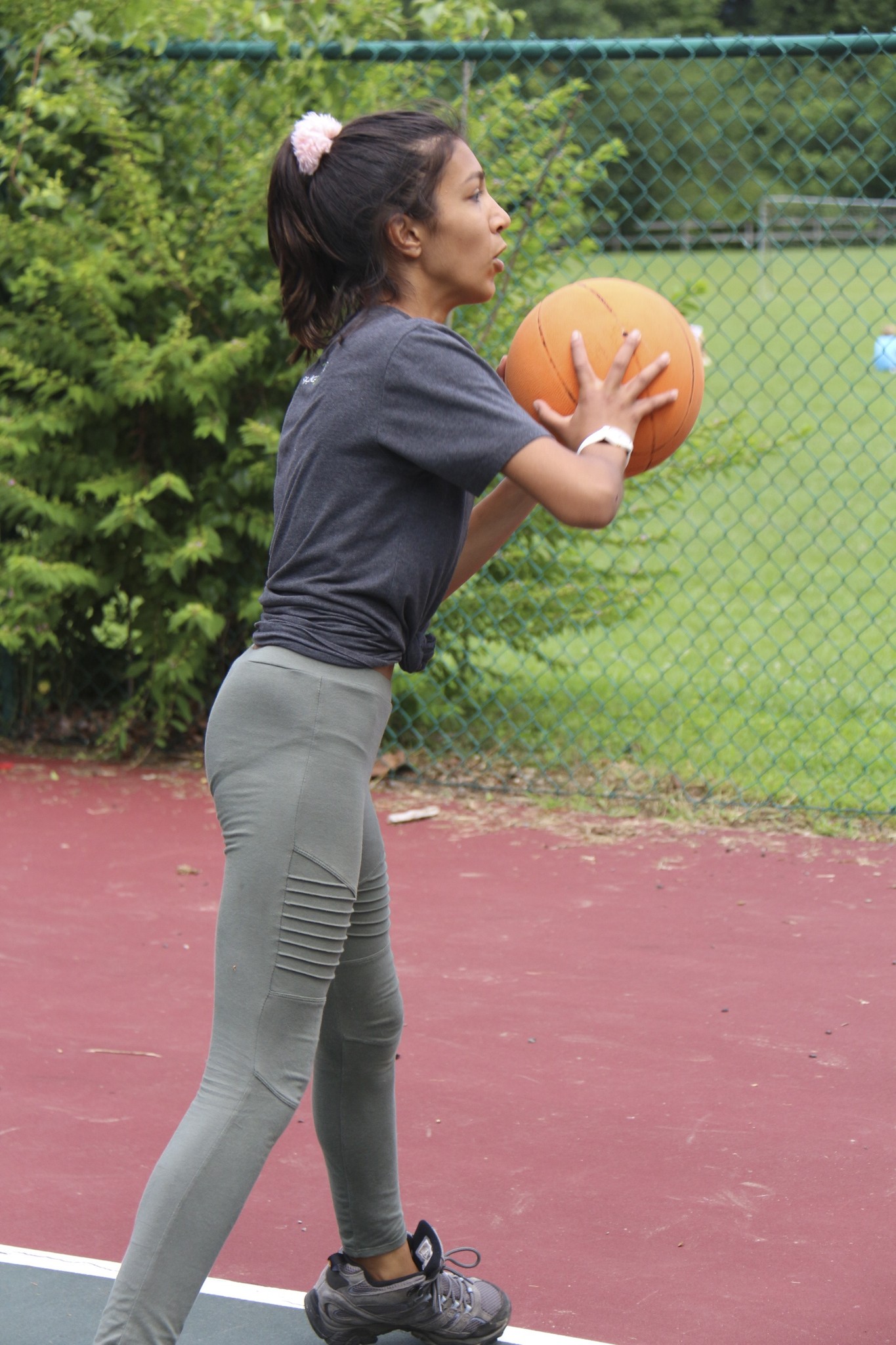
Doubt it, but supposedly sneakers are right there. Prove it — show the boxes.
[304,1220,512,1345]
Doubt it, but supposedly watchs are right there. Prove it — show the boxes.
[577,426,635,469]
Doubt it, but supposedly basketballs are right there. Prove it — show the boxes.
[506,277,705,478]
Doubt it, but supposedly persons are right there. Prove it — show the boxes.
[94,112,712,1345]
